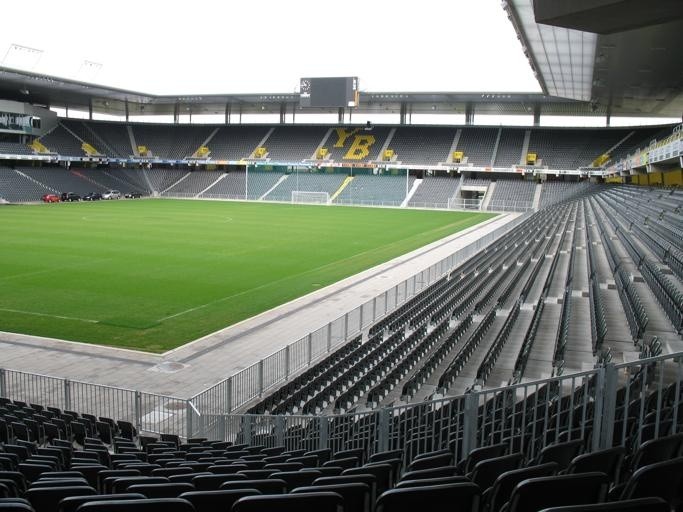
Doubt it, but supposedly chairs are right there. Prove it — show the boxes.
[0,119,602,214]
[0,394,682,512]
[273,182,681,394]
